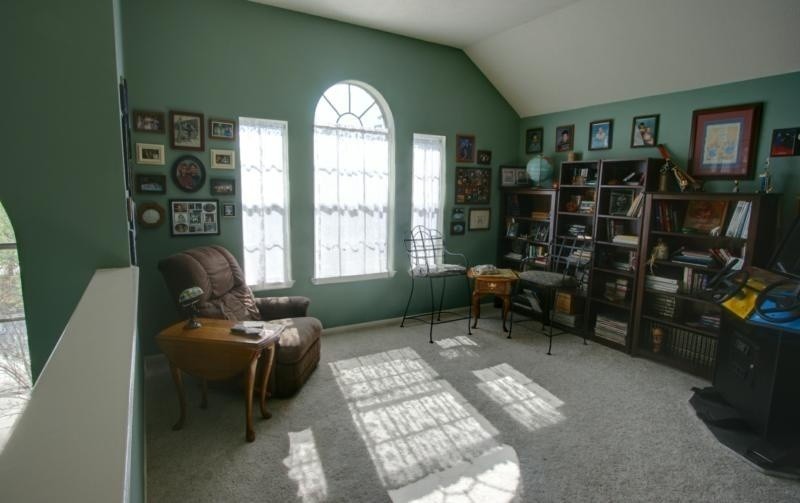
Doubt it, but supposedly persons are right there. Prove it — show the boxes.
[177,164,189,187]
[189,164,201,188]
[595,127,606,147]
[638,122,653,145]
[529,133,540,151]
[558,131,571,150]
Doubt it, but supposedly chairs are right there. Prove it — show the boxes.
[400,225,468,344]
[507,227,594,356]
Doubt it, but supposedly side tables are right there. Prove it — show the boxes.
[467,265,519,333]
[154,317,284,442]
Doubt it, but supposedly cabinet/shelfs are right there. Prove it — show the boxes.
[542,162,600,341]
[628,192,781,374]
[493,189,558,327]
[588,157,668,356]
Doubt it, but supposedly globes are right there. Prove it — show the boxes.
[526,154,554,190]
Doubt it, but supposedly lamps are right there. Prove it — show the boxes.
[179,286,205,330]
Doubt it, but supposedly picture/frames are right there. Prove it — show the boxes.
[630,112,660,149]
[770,127,799,157]
[221,203,236,218]
[211,179,237,196]
[455,166,491,205]
[130,232,136,265]
[136,173,167,194]
[120,82,129,113]
[555,124,574,153]
[587,118,611,151]
[135,142,165,165]
[169,109,205,152]
[525,128,544,154]
[499,165,532,188]
[686,101,760,180]
[128,197,133,221]
[210,148,235,170]
[478,150,492,164]
[453,208,466,219]
[170,199,221,236]
[468,208,491,231]
[123,116,132,189]
[451,222,465,234]
[172,154,205,192]
[133,110,165,133]
[454,133,475,163]
[210,118,235,141]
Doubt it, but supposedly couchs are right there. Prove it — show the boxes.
[159,242,321,399]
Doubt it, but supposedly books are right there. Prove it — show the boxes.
[641,321,719,369]
[555,265,588,295]
[653,201,751,239]
[531,211,551,219]
[594,312,628,347]
[603,279,632,304]
[510,287,545,314]
[564,248,593,265]
[550,294,584,330]
[649,236,747,270]
[646,293,722,333]
[565,193,596,215]
[597,248,638,273]
[645,264,734,304]
[521,244,548,264]
[505,222,550,242]
[600,219,639,245]
[604,170,645,185]
[566,225,592,240]
[573,168,598,185]
[609,189,646,217]
